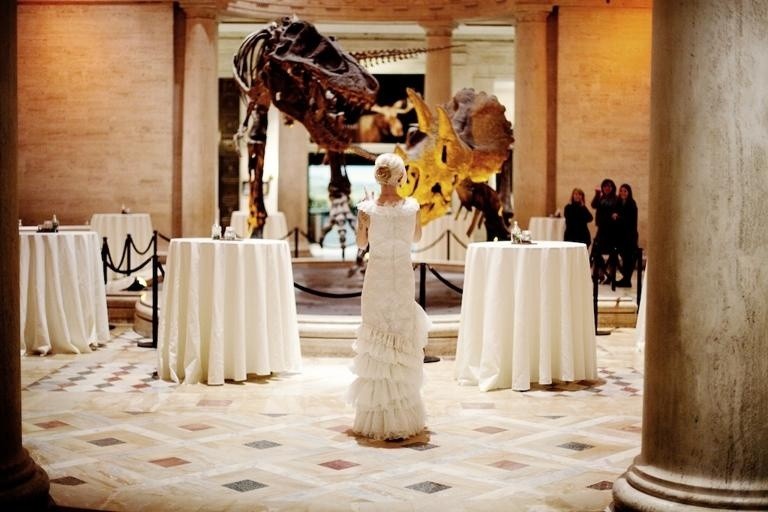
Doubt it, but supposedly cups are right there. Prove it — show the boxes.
[521,230,531,241]
[211,223,222,239]
[226,227,235,240]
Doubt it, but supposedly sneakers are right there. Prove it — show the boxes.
[591,270,633,289]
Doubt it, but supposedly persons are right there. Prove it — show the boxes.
[590,178,618,285]
[610,183,640,288]
[562,187,593,249]
[343,152,433,440]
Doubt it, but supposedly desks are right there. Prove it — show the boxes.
[421,213,599,393]
[228,212,289,241]
[89,213,156,289]
[158,236,302,384]
[18,223,110,356]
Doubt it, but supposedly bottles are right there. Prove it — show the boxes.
[511,220,521,244]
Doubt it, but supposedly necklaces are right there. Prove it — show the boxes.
[379,193,400,198]
